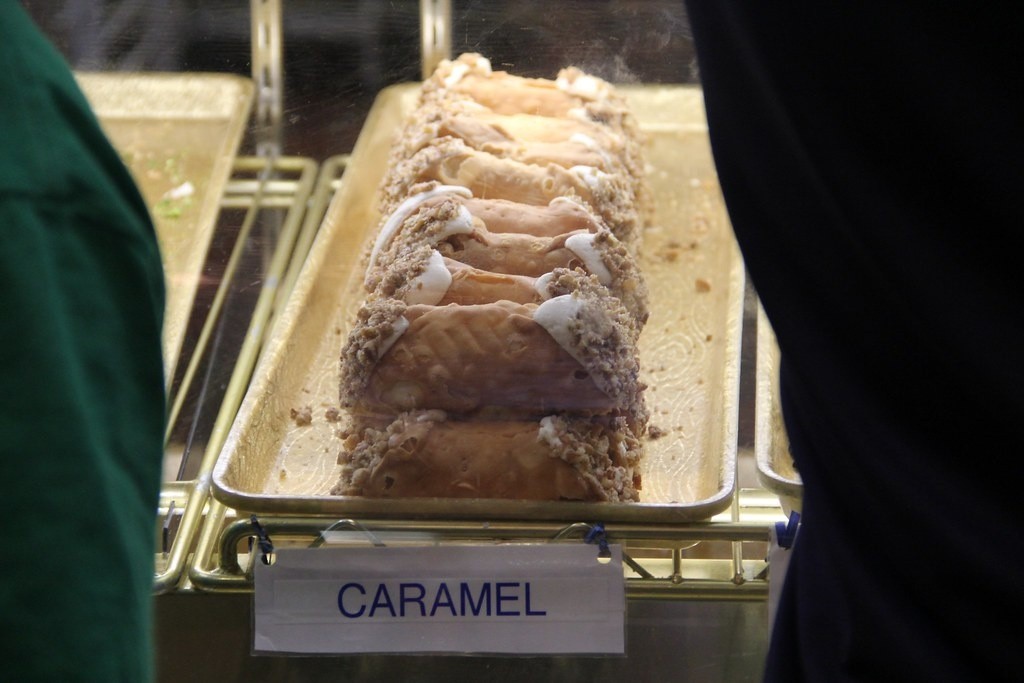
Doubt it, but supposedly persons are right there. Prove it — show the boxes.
[0,1,171,683]
[683,1,1024,683]
